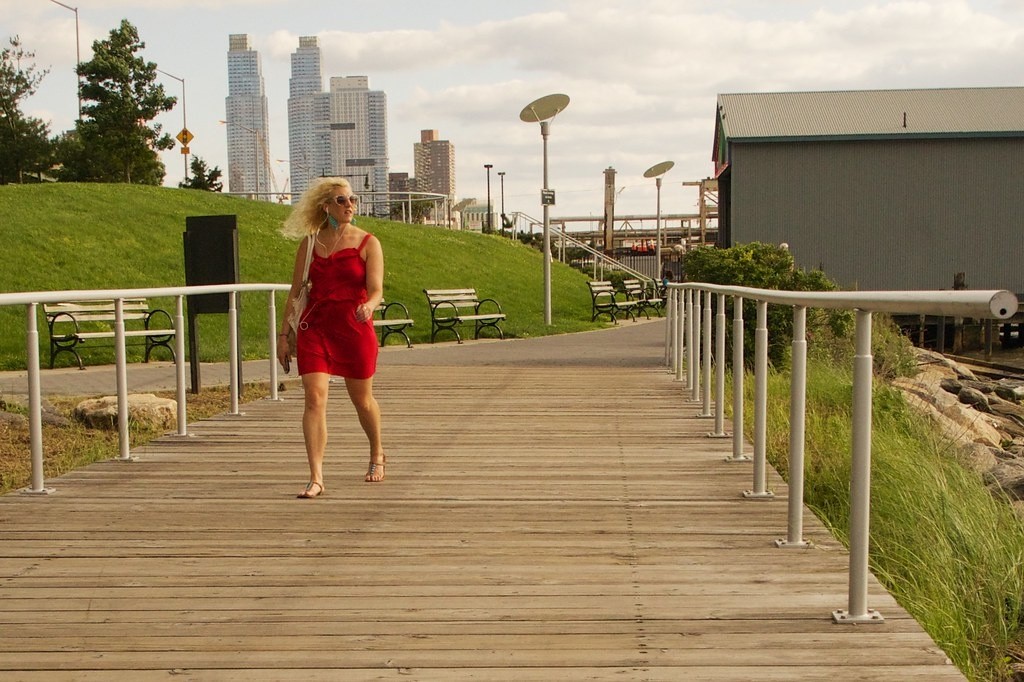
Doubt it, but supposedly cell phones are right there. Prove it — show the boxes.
[283,356,289,374]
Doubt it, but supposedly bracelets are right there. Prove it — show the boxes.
[279,334,289,338]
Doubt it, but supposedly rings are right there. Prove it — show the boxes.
[363,307,367,310]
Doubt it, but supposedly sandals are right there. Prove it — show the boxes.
[365,453,386,482]
[297,481,325,498]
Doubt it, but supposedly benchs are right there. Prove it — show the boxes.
[423,288,507,345]
[42,299,176,370]
[586,280,637,325]
[373,298,415,349]
[623,280,663,320]
[653,278,667,308]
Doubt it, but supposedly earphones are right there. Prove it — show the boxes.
[326,207,328,213]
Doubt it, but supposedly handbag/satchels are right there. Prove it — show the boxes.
[287,233,316,355]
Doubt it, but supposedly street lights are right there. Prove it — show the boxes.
[277,160,311,193]
[643,161,674,303]
[219,119,259,203]
[320,168,370,189]
[519,93,570,327]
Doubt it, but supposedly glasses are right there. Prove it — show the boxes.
[333,194,359,206]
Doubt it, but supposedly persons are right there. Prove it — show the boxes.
[277,177,387,499]
